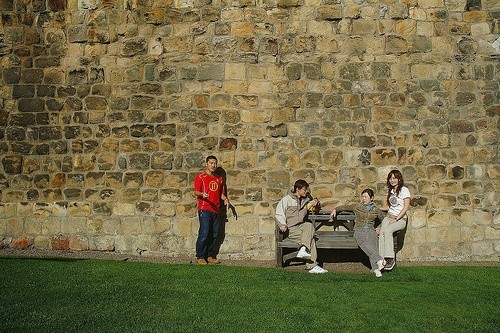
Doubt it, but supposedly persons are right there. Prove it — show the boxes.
[193,152,233,267]
[325,190,391,279]
[378,170,410,271]
[274,177,331,277]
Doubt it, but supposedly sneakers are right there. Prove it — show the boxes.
[384,258,395,270]
[376,260,387,270]
[296,251,312,259]
[373,270,382,277]
[308,266,329,274]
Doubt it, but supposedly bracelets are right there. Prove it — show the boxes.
[394,218,398,222]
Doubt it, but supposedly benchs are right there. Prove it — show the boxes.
[274,209,398,268]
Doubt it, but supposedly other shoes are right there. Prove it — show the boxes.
[206,257,219,264]
[197,258,207,265]
